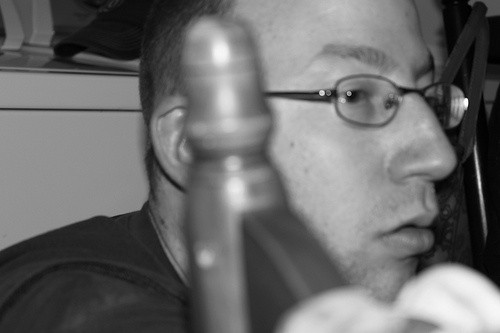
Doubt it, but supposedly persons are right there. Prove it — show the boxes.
[0,0,456,333]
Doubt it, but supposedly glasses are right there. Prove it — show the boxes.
[262,73,469,129]
